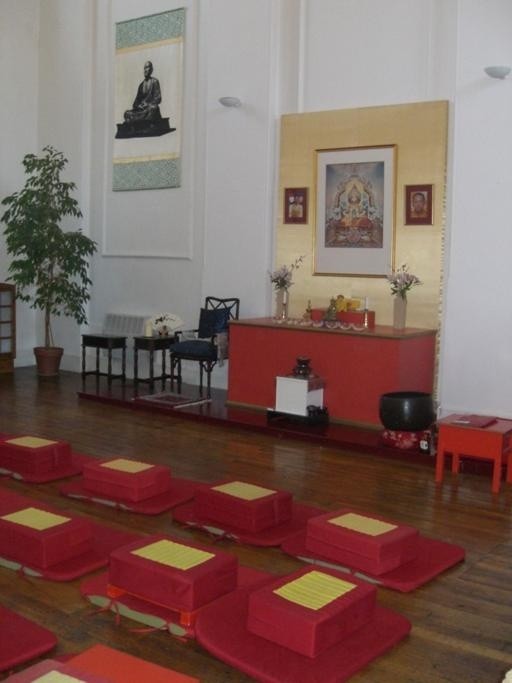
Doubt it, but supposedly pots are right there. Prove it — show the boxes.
[378,390,436,431]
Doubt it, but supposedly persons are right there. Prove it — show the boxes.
[411,192,428,218]
[289,193,302,217]
[333,174,377,220]
[124,60,161,122]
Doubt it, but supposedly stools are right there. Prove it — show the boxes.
[81,333,127,387]
[132,335,181,393]
[434,414,512,493]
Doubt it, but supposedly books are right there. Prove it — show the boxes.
[452,412,498,429]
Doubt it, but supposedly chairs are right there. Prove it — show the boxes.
[170,297,239,400]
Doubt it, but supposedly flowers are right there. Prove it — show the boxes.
[263,254,309,288]
[386,264,424,300]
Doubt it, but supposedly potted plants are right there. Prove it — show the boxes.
[0,145,95,376]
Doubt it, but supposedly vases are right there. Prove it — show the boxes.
[392,293,407,330]
[273,287,288,321]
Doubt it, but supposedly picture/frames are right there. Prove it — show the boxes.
[310,144,397,278]
[282,186,310,227]
[403,183,435,226]
